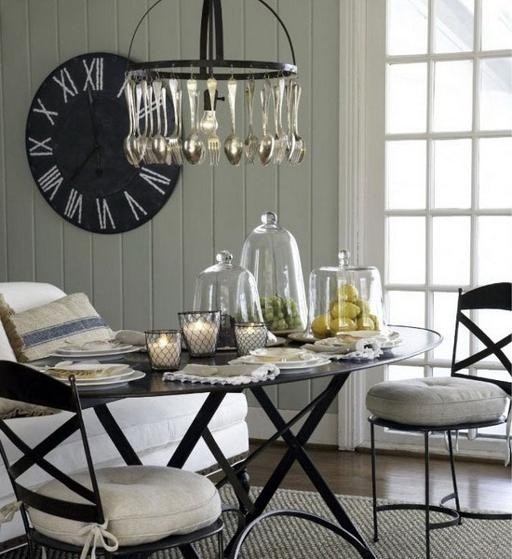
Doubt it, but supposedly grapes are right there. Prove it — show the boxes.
[237,294,302,330]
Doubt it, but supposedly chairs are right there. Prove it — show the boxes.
[361,282,511,557]
[2,359,229,558]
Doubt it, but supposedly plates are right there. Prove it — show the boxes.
[228,347,332,374]
[50,344,144,359]
[337,330,405,350]
[43,363,147,387]
[181,337,288,352]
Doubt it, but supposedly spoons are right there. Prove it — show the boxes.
[183,78,202,166]
[223,77,243,166]
[122,78,183,167]
[244,77,305,168]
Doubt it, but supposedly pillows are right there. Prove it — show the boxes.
[0,291,115,371]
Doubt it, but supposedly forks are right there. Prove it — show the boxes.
[207,78,221,167]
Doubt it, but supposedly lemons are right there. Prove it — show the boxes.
[311,283,377,339]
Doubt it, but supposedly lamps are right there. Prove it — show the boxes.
[117,1,311,172]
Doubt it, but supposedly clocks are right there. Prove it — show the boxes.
[24,51,187,234]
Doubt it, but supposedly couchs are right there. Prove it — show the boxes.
[1,279,249,546]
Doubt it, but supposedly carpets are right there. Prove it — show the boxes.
[7,476,512,556]
